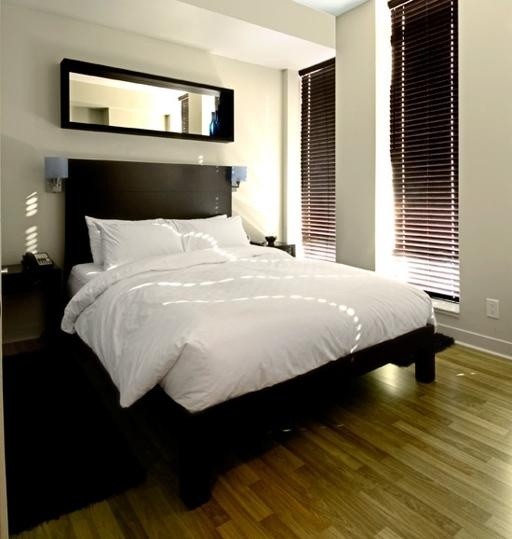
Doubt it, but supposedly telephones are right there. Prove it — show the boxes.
[24,252,54,266]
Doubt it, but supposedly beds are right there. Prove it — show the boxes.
[65,157,454,513]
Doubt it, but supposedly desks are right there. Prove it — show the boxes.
[2,262,65,348]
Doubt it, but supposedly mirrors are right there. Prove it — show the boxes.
[60,57,235,143]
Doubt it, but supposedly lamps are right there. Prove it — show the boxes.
[44,156,69,192]
[231,165,248,188]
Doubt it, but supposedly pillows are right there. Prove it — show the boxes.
[84,214,251,272]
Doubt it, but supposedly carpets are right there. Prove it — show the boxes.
[2,346,305,536]
